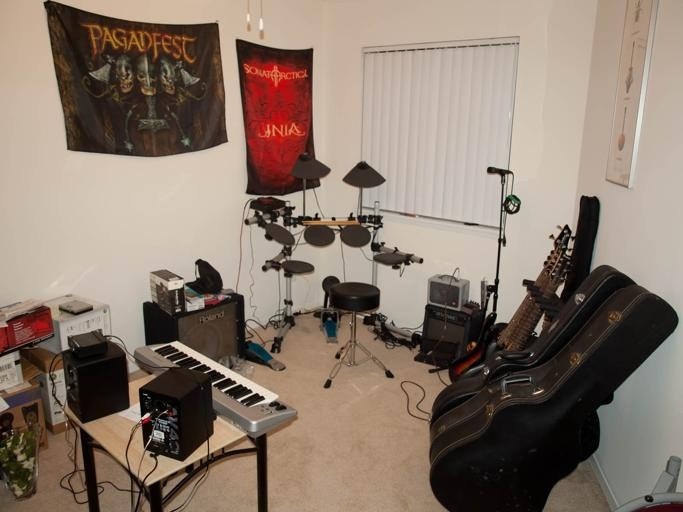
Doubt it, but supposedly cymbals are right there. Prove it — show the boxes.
[343,162,385,187]
[290,154,330,179]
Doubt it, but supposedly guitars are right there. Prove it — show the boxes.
[449,224,576,383]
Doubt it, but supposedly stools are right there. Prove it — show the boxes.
[323,282,395,387]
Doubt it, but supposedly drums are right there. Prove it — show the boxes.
[266,224,294,244]
[341,226,371,246]
[374,252,405,264]
[282,261,314,273]
[304,225,335,246]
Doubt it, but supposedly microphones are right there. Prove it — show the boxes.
[487,166,510,174]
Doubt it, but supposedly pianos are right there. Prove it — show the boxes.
[134,339,297,439]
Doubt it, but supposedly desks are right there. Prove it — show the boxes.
[61,371,268,512]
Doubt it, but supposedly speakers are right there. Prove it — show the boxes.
[420,302,484,361]
[138,366,214,462]
[61,340,130,424]
[142,292,247,368]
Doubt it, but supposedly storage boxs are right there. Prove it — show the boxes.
[0,294,112,454]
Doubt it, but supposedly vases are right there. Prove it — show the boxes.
[605,0,659,188]
